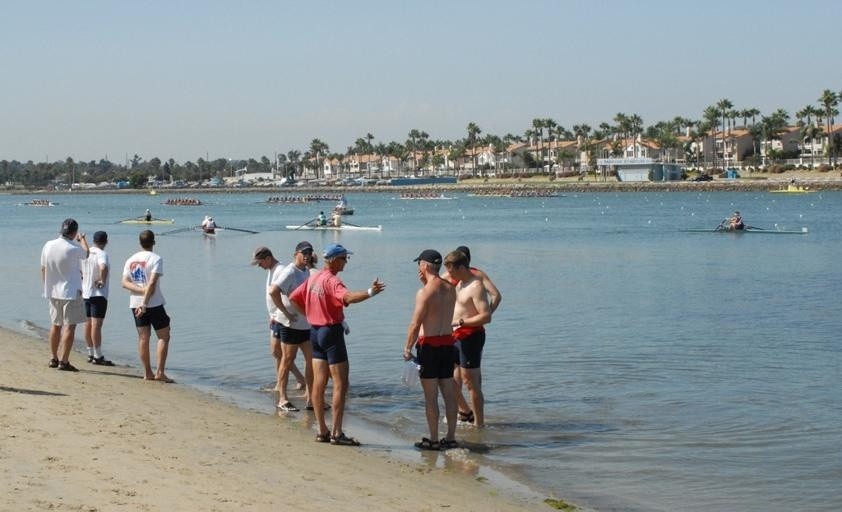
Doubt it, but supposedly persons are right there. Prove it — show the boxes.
[270,241,332,411]
[726,211,744,231]
[440,246,501,422]
[444,250,491,431]
[404,249,458,450]
[121,230,173,383]
[143,208,151,221]
[289,244,387,446]
[341,194,347,206]
[316,211,326,225]
[290,251,334,379]
[41,218,90,371]
[204,217,216,233]
[81,231,115,366]
[332,212,341,227]
[252,247,306,390]
[201,215,209,225]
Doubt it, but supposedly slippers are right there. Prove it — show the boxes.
[305,399,331,410]
[276,401,300,412]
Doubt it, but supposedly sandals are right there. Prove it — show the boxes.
[93,356,109,365]
[439,437,458,449]
[58,361,78,372]
[49,358,59,368]
[88,355,94,362]
[328,432,358,446]
[414,437,441,451]
[458,409,475,423]
[315,429,330,443]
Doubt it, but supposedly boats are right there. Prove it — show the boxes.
[677,227,812,235]
[17,187,568,239]
[767,184,818,192]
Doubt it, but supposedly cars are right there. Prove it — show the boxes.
[688,174,714,182]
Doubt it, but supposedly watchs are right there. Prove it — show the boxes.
[459,319,464,326]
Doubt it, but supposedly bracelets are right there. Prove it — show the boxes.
[141,303,147,307]
[367,287,376,297]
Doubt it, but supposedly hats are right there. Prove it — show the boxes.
[94,231,108,243]
[413,249,442,264]
[58,218,79,233]
[322,243,354,258]
[296,240,314,251]
[250,246,272,265]
